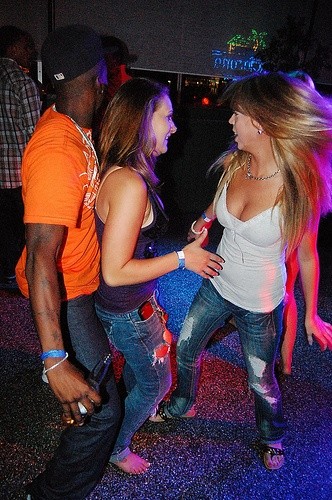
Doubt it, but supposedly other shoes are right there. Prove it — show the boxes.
[0,272,21,290]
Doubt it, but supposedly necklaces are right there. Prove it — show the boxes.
[243,153,281,181]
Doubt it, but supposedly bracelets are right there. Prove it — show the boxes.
[41,352,69,384]
[191,220,206,235]
[201,212,212,223]
[174,250,186,270]
[40,350,67,360]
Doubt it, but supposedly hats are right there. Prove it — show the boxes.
[39,23,119,84]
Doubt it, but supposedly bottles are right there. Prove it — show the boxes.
[69,353,112,426]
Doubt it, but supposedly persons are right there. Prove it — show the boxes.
[93,36,133,142]
[147,71,332,470]
[0,25,42,280]
[14,24,124,500]
[94,77,225,475]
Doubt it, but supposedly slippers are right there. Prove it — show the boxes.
[149,400,197,422]
[261,445,285,470]
